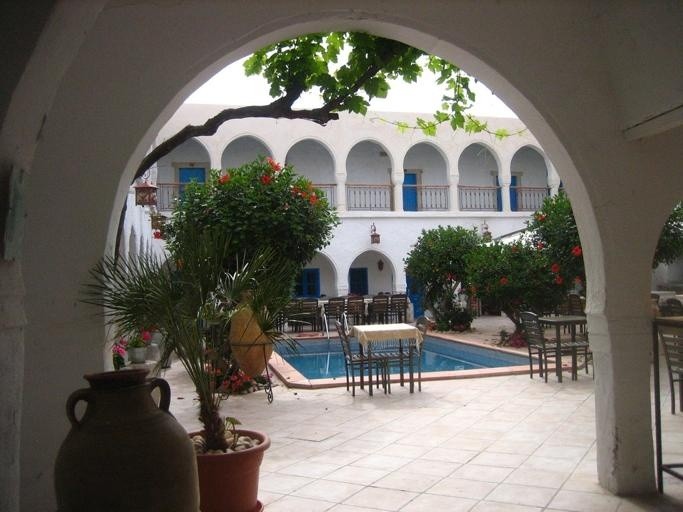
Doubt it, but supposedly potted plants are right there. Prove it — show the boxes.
[74,211,322,512]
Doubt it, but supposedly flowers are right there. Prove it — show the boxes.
[153,152,343,289]
[111,330,153,371]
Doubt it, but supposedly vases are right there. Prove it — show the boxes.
[52,367,202,512]
[228,282,274,379]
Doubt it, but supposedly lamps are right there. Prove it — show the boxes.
[481,219,493,243]
[149,210,168,231]
[370,221,381,245]
[132,168,160,208]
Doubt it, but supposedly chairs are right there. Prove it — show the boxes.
[518,291,683,416]
[281,291,408,333]
[334,314,428,398]
